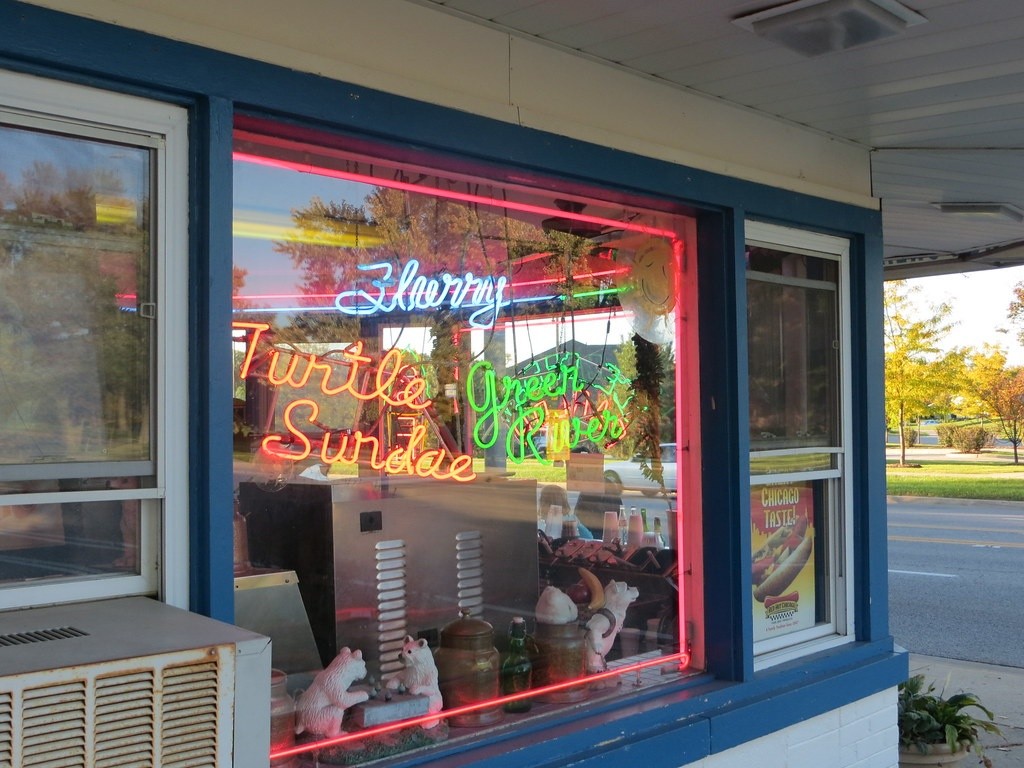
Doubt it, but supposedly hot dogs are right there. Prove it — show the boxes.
[750,514,812,602]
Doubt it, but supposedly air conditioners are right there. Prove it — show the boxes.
[0,594,272,767]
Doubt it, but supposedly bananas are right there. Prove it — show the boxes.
[579,567,604,610]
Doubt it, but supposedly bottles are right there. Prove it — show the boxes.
[618,508,627,547]
[641,508,649,532]
[501,614,535,714]
[653,516,665,549]
[602,511,619,546]
[630,507,637,516]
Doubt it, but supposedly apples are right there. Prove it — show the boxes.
[566,584,590,603]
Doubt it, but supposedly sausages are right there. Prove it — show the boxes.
[764,592,799,607]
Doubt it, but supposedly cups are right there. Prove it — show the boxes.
[544,503,563,538]
[628,516,643,547]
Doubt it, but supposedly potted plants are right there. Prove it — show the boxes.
[897,669,1010,768]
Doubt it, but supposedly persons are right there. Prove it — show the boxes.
[538,484,593,540]
[574,469,623,539]
